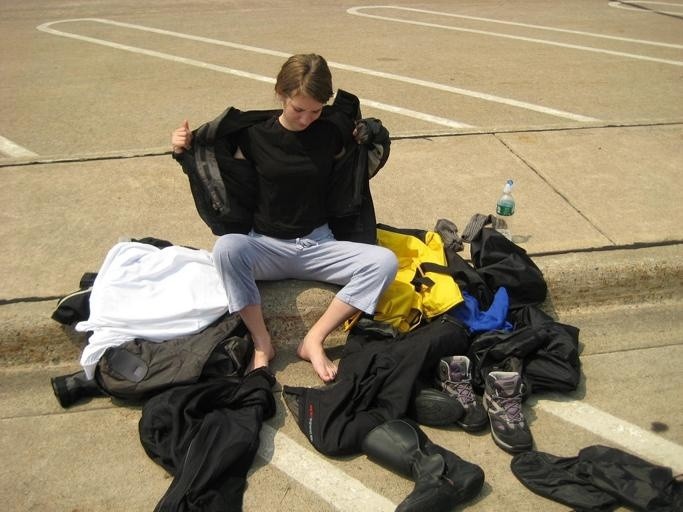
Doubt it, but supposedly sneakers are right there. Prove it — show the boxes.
[482,371,533,454]
[437,355,489,432]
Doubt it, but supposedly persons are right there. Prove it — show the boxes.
[172,54,391,382]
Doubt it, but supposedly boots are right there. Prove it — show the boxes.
[360,417,484,512]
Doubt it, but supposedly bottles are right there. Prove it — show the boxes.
[491,181,516,242]
